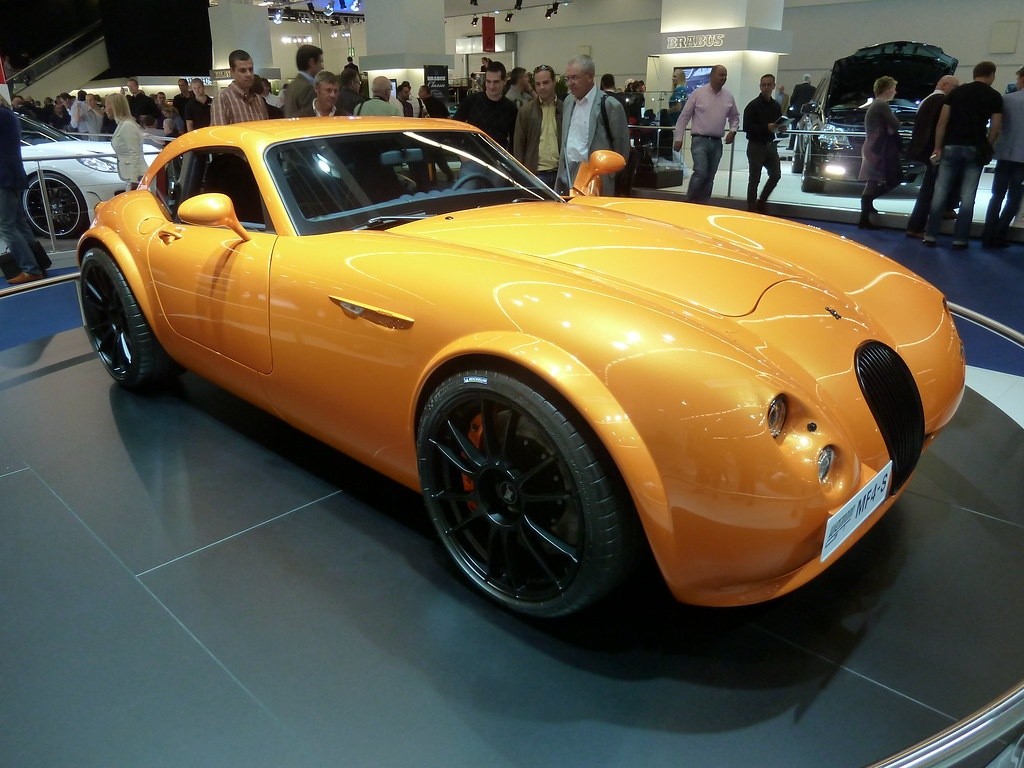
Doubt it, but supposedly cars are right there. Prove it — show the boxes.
[791,40,961,194]
[13,108,165,241]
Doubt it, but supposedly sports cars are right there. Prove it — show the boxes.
[75,114,967,631]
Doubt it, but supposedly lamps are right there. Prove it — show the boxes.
[470,0,478,6]
[554,2,561,14]
[505,12,514,22]
[262,0,366,26]
[545,10,553,20]
[471,17,479,27]
[514,0,524,10]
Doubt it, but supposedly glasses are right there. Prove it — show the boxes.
[354,81,361,85]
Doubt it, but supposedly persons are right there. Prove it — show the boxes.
[10,78,212,181]
[105,93,148,192]
[672,65,740,202]
[775,85,790,137]
[855,77,904,229]
[207,44,457,187]
[743,73,788,210]
[905,62,1024,248]
[668,68,685,108]
[450,51,629,201]
[785,75,817,150]
[3,51,30,95]
[624,78,646,92]
[0,92,48,284]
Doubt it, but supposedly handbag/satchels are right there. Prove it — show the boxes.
[419,98,430,118]
[871,128,904,156]
[786,104,801,118]
[614,164,635,194]
[0,240,53,280]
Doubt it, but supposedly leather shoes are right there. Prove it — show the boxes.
[7,271,43,284]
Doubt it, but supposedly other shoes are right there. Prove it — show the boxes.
[786,147,793,150]
[905,229,924,238]
[923,234,937,245]
[942,210,958,219]
[952,241,970,248]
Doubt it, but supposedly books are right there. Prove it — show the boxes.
[774,116,795,134]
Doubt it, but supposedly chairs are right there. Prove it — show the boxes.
[195,156,259,219]
[334,142,408,205]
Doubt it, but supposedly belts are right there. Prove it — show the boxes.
[692,134,721,140]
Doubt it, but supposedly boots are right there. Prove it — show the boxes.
[857,195,879,229]
[869,185,886,212]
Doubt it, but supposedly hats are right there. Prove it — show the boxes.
[625,79,634,88]
